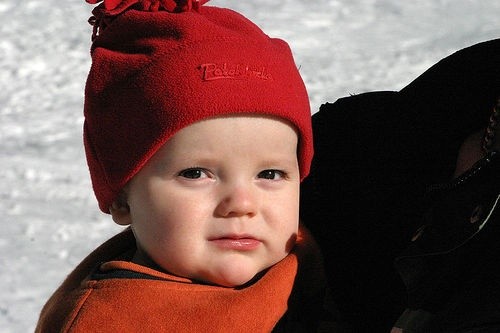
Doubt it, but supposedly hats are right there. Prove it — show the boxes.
[84,0,315,212]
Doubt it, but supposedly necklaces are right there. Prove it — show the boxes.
[479,92,500,202]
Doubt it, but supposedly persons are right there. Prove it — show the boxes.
[32,0,314,333]
[270,38,500,333]
